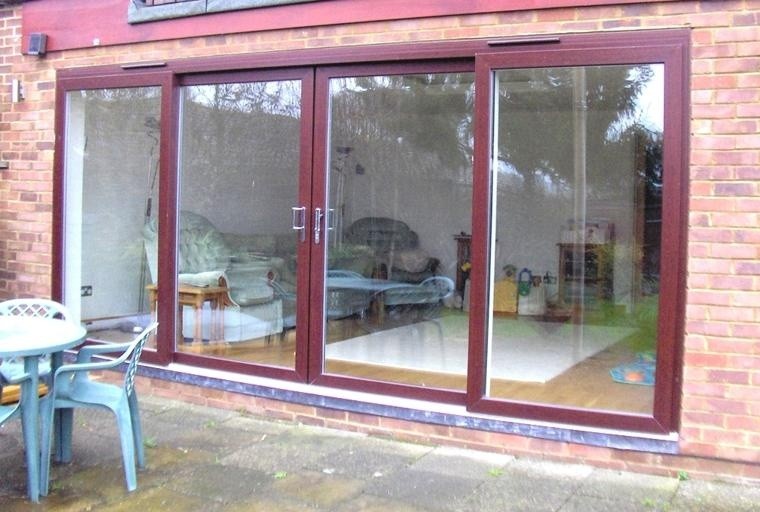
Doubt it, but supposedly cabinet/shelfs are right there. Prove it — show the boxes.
[556,242,614,320]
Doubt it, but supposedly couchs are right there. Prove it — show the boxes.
[223,233,375,333]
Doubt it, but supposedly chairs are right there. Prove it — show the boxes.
[0,299,159,504]
[145,209,284,350]
[345,217,455,322]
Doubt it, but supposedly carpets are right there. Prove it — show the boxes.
[323,315,640,384]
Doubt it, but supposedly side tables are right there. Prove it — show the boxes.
[147,284,231,354]
